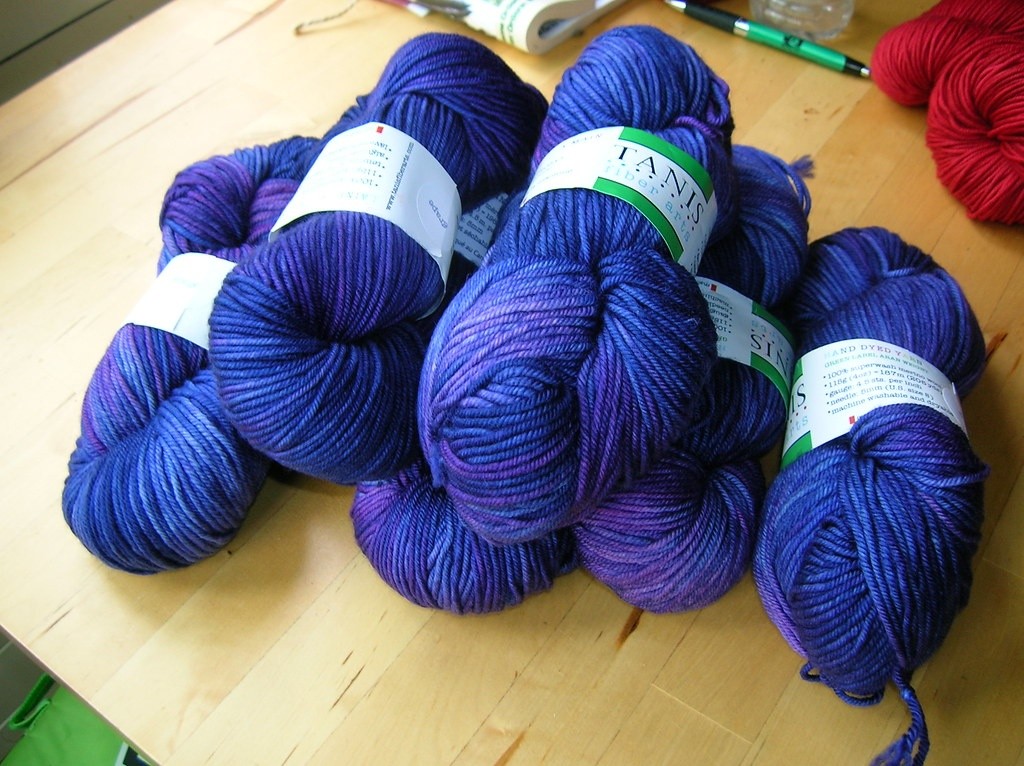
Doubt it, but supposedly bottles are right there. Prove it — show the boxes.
[750,0,854,40]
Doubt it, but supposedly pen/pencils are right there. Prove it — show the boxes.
[663,0,871,78]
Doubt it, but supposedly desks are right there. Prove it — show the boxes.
[0,0,1024,766]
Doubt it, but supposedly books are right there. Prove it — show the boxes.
[415,0,626,55]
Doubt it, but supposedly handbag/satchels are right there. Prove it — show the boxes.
[0,674,151,766]
